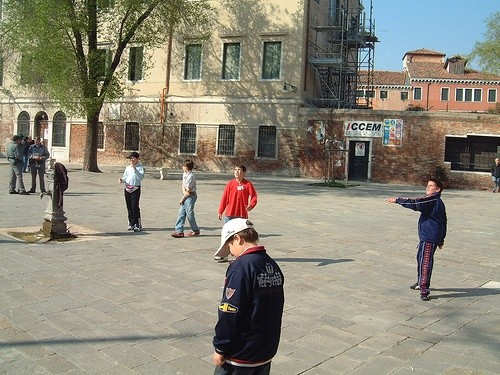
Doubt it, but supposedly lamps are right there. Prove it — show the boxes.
[282,83,296,92]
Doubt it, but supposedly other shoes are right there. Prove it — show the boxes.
[134,225,143,232]
[410,283,420,289]
[421,295,430,301]
[215,256,228,261]
[20,191,31,195]
[171,232,184,238]
[187,230,201,236]
[127,225,133,230]
[9,191,19,194]
[29,188,35,193]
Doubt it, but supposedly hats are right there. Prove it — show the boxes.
[214,218,254,257]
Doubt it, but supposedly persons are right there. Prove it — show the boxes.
[352,15,358,34]
[214,164,258,260]
[490,157,500,193]
[212,218,284,375]
[27,136,50,193]
[6,135,30,195]
[17,133,35,173]
[170,160,200,238]
[385,178,447,301]
[119,152,144,232]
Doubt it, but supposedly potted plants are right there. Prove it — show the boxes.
[433,165,450,189]
